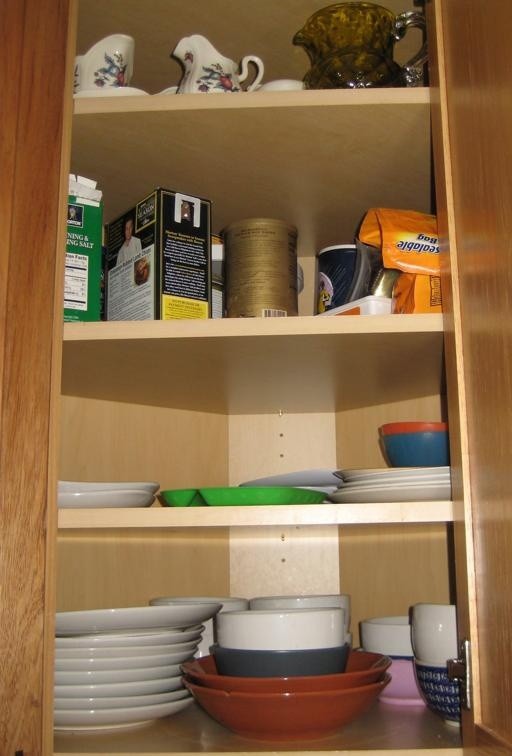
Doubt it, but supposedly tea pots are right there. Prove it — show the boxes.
[292,3,426,90]
[74,33,152,96]
[155,87,179,95]
[248,78,303,92]
[172,34,264,93]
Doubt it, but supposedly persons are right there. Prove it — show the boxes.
[115,216,143,267]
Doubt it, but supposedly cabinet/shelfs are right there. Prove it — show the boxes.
[1,0,511,755]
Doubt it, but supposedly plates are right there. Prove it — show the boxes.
[177,652,393,740]
[157,470,337,508]
[334,465,453,503]
[53,601,223,734]
[55,478,157,508]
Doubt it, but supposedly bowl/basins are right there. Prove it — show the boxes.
[379,421,449,468]
[149,593,352,674]
[359,600,461,730]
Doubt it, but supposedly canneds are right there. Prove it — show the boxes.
[211,230,227,318]
[317,243,380,313]
